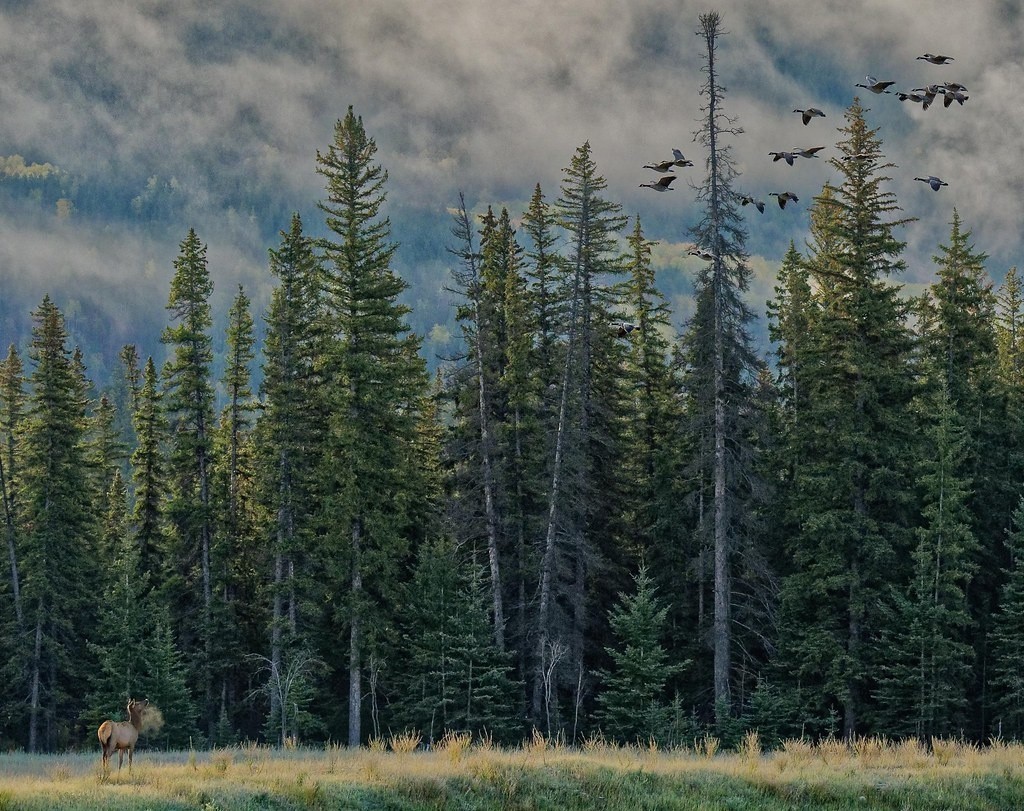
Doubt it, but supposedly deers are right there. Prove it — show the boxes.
[97,698,149,774]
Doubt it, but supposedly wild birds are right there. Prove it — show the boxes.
[937,81,968,92]
[768,151,798,166]
[661,148,694,167]
[916,53,955,64]
[854,75,895,94]
[638,176,677,192]
[793,107,826,125]
[911,85,946,111]
[913,175,948,192]
[792,146,825,159]
[643,161,676,173]
[894,91,925,103]
[938,90,969,108]
[735,193,766,214]
[768,191,800,210]
